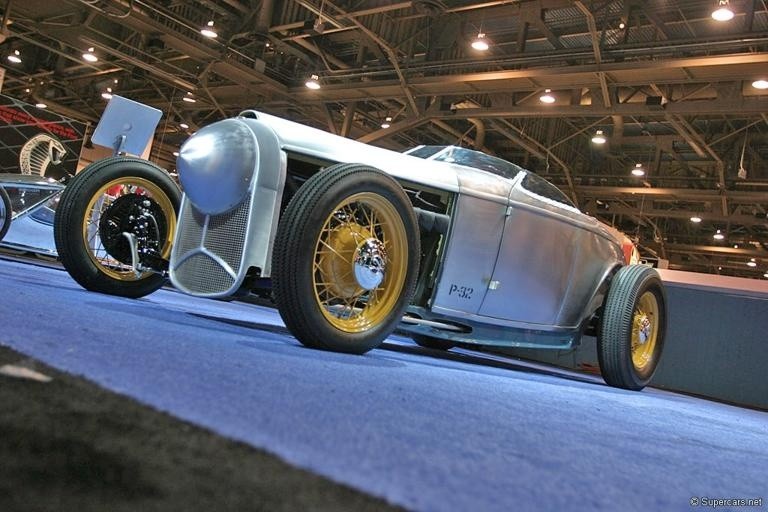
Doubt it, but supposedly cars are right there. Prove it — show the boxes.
[54,94,670,389]
[0,172,67,262]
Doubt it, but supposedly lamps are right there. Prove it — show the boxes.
[81,45,102,64]
[629,163,646,179]
[179,118,191,131]
[7,46,24,66]
[100,84,115,101]
[199,1,220,40]
[172,147,179,159]
[181,89,198,104]
[378,114,394,130]
[591,128,606,146]
[711,229,726,243]
[470,1,492,52]
[688,212,703,225]
[35,102,47,109]
[303,55,324,91]
[749,76,768,92]
[762,270,767,278]
[537,88,557,104]
[710,1,737,23]
[744,258,758,270]
